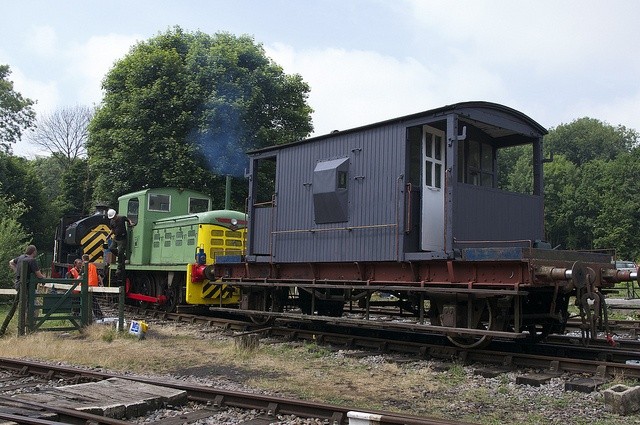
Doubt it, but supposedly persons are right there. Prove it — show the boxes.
[9,245,45,332]
[79,254,105,320]
[66,258,82,318]
[105,209,133,276]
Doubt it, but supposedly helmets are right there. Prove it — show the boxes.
[108,208,116,219]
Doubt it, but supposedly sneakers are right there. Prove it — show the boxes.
[124,257,127,260]
[115,269,121,274]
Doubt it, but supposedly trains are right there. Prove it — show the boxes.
[52,101,640,348]
